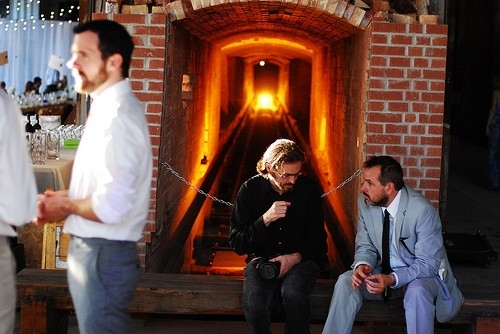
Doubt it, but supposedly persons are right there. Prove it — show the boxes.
[37,19,153,334]
[0,74,67,334]
[322,156,465,334]
[228,139,328,334]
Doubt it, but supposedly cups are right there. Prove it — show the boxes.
[12,93,57,107]
[25,124,84,165]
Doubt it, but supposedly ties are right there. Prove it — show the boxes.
[381,209,391,304]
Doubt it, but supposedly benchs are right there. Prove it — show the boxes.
[13,268,500,334]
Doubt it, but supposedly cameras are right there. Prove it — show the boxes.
[256,253,282,279]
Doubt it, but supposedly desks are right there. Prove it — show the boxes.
[33,148,77,194]
[20,102,74,124]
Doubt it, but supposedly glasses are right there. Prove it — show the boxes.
[273,166,303,178]
